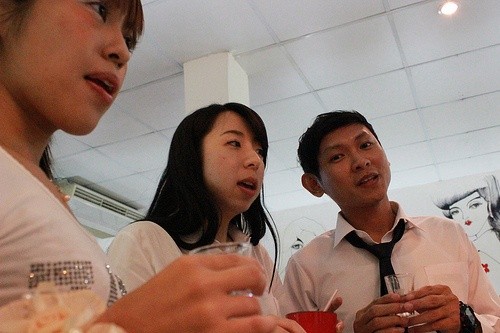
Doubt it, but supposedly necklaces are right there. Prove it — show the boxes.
[48,180,70,202]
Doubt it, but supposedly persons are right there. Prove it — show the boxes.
[107,102,344,333]
[0,0,276,333]
[282,110,500,333]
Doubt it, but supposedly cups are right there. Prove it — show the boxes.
[285,311,338,333]
[190,243,255,300]
[383,273,416,318]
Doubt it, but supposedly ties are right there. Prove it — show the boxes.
[345,219,405,297]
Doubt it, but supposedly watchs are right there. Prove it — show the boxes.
[436,300,475,333]
